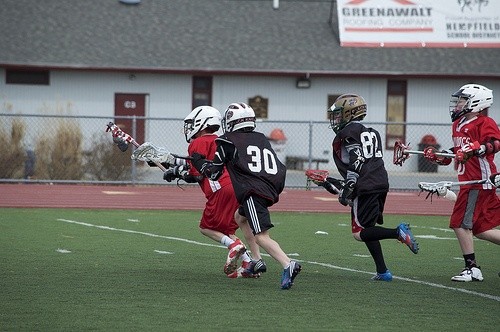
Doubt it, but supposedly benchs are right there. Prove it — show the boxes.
[284,156,330,170]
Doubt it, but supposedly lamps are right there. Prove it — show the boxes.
[297,77,312,88]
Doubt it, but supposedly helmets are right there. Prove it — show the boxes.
[184,106,221,142]
[449,84,493,122]
[328,94,367,133]
[221,103,256,133]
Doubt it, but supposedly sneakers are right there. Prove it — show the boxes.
[227,260,260,279]
[242,259,267,274]
[370,270,392,281]
[280,260,302,290]
[451,266,484,282]
[224,238,247,275]
[397,223,419,254]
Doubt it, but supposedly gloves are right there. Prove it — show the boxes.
[323,178,345,194]
[148,160,170,169]
[424,146,452,166]
[163,166,179,182]
[338,179,360,206]
[454,141,481,170]
[191,153,206,173]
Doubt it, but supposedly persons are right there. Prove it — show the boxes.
[190,102,301,289]
[424,84,500,282]
[305,93,419,281]
[131,106,260,278]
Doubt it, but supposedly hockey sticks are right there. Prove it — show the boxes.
[416,178,490,204]
[129,141,214,165]
[304,168,355,208]
[392,139,468,168]
[105,120,176,181]
